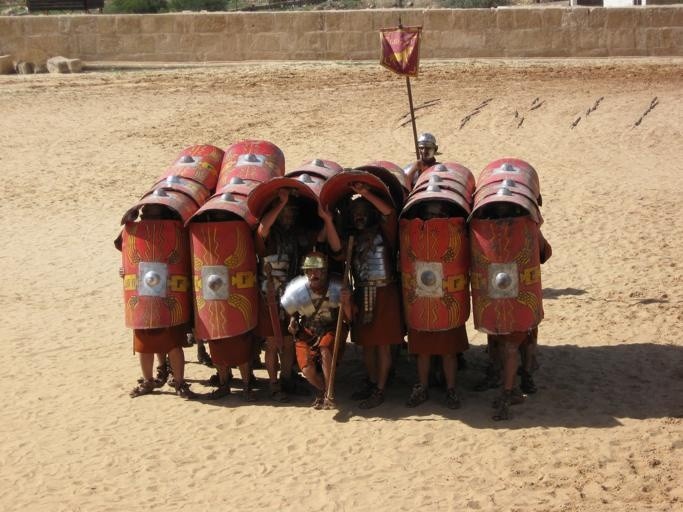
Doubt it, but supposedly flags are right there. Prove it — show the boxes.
[376,25,423,79]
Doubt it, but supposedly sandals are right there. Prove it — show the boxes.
[350,340,540,422]
[129,349,338,410]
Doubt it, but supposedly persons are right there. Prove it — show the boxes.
[110,178,553,422]
[403,131,443,186]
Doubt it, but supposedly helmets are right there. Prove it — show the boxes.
[301,252,329,270]
[417,134,437,149]
